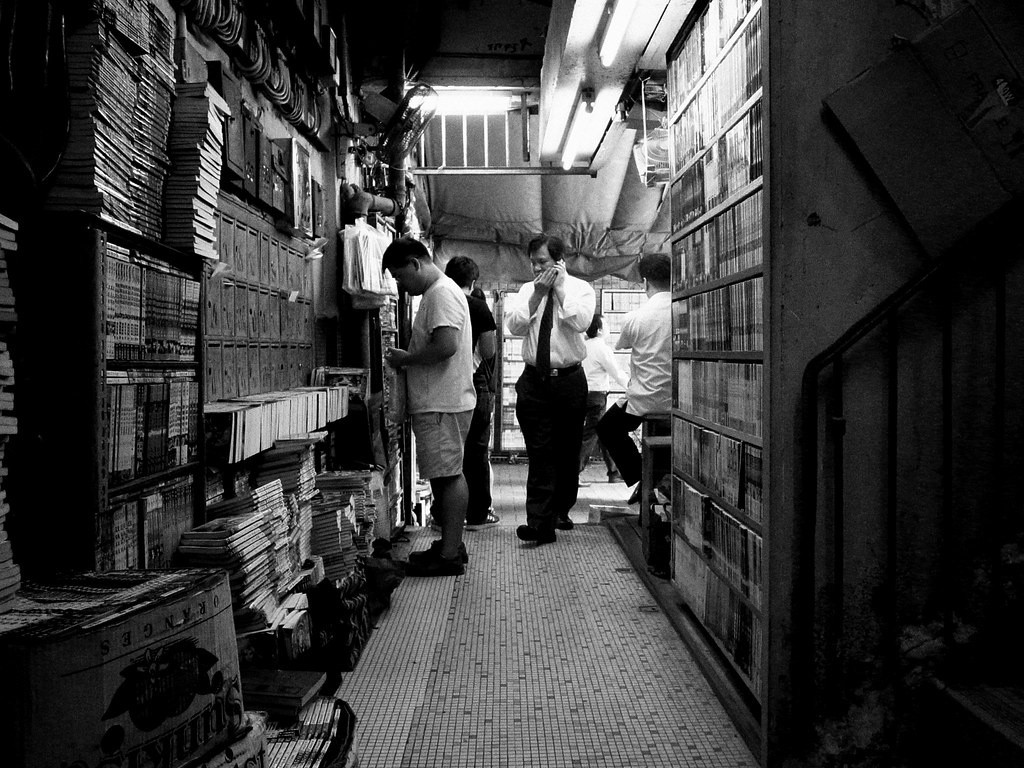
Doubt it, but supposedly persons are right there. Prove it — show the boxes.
[430,256,496,530]
[506,234,596,542]
[577,314,631,487]
[384,237,476,577]
[615,254,671,505]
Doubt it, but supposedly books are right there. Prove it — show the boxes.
[667,0,763,703]
[0,213,21,615]
[44,0,380,714]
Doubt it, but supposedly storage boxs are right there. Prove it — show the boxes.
[824,6,1023,257]
[0,572,253,768]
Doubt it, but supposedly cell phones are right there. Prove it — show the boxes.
[555,259,564,276]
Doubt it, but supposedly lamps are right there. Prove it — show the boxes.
[559,86,598,170]
[598,0,637,70]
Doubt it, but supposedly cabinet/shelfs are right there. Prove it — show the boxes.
[666,0,1024,767]
[0,0,382,768]
[411,284,648,466]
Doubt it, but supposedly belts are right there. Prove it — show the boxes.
[525,362,581,376]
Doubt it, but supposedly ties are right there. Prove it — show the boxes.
[536,288,554,381]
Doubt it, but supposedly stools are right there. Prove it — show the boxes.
[639,412,669,577]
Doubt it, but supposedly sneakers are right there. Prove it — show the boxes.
[431,519,442,532]
[405,538,468,577]
[466,513,500,531]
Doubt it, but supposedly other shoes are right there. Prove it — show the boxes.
[516,524,556,542]
[627,482,639,505]
[556,518,574,530]
